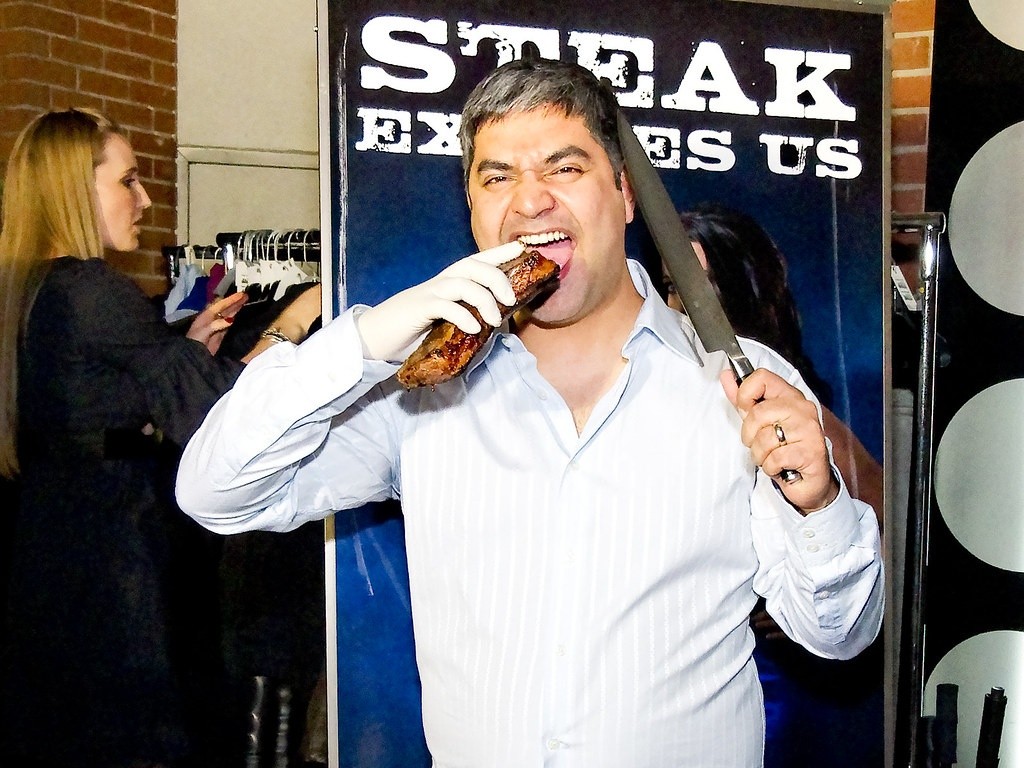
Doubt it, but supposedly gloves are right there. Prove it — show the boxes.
[354,241,525,365]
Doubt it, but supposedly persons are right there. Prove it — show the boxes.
[0,107,321,768]
[176,56,886,767]
[661,211,883,767]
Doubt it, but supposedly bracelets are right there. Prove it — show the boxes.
[262,329,291,343]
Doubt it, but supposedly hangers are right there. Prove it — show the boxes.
[163,227,320,282]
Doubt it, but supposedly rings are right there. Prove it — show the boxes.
[774,422,788,447]
[217,312,223,318]
